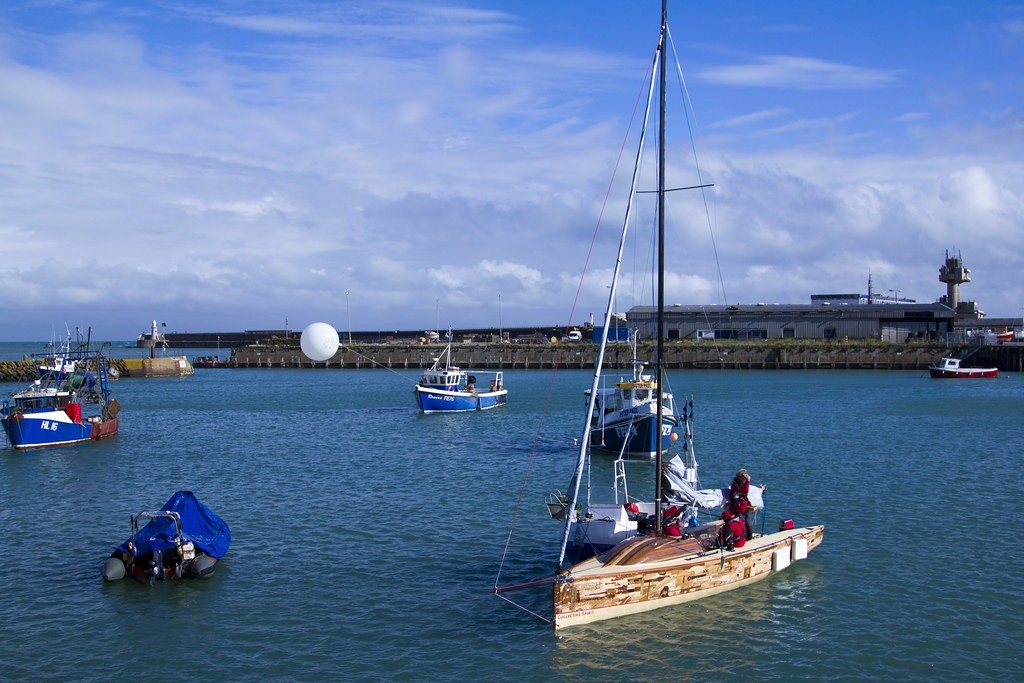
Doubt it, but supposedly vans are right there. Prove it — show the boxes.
[568,330,583,343]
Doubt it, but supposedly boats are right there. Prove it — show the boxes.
[0,322,123,449]
[582,329,679,460]
[974,326,1014,342]
[101,491,230,588]
[928,357,998,378]
[193,335,227,368]
[414,324,507,414]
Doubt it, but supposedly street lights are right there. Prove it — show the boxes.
[497,292,503,343]
[889,289,902,302]
[345,291,353,345]
[606,285,620,341]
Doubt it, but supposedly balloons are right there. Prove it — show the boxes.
[301,322,343,360]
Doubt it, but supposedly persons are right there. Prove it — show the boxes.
[660,462,689,531]
[724,468,755,540]
[715,512,748,551]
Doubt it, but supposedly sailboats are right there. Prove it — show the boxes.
[494,0,826,633]
